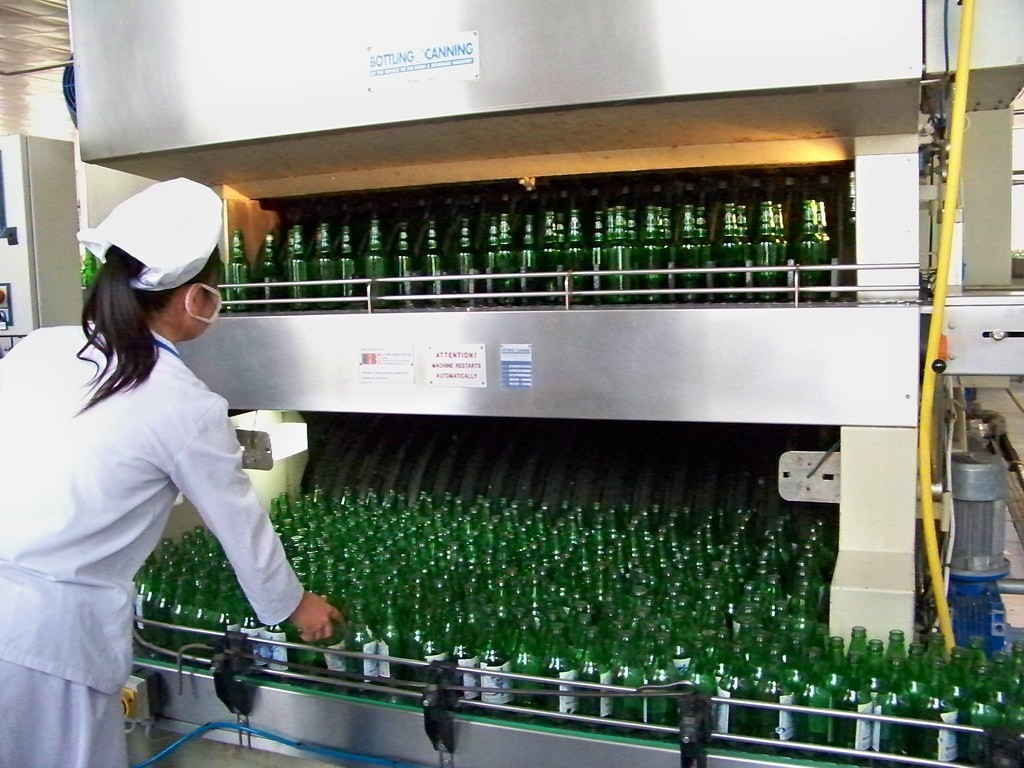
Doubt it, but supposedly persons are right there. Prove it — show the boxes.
[0,177,345,768]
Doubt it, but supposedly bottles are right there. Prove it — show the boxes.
[81,246,101,303]
[127,484,1024,768]
[1011,249,1024,257]
[218,171,856,312]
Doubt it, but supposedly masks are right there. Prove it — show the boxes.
[185,283,223,328]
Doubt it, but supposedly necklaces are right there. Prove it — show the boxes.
[151,339,179,358]
